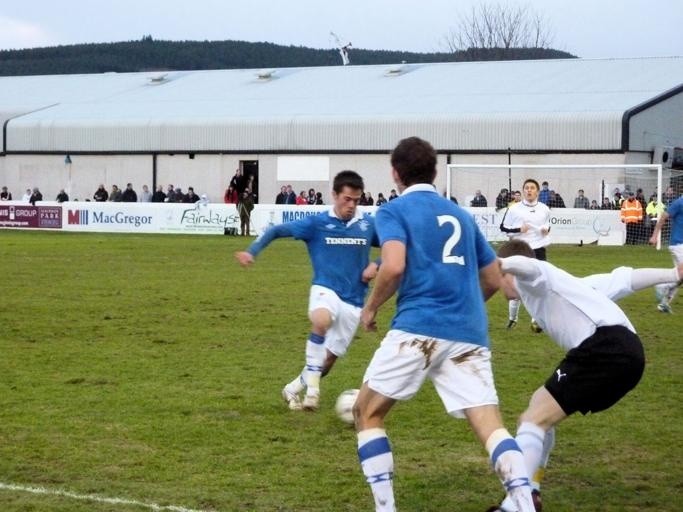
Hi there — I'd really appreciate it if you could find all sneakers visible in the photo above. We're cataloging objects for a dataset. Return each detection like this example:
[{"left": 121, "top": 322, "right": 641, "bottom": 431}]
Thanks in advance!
[
  {"left": 656, "top": 302, "right": 673, "bottom": 313},
  {"left": 486, "top": 488, "right": 543, "bottom": 512},
  {"left": 530, "top": 322, "right": 542, "bottom": 334},
  {"left": 281, "top": 383, "right": 303, "bottom": 411},
  {"left": 654, "top": 282, "right": 672, "bottom": 304},
  {"left": 506, "top": 320, "right": 517, "bottom": 330},
  {"left": 303, "top": 392, "right": 320, "bottom": 411}
]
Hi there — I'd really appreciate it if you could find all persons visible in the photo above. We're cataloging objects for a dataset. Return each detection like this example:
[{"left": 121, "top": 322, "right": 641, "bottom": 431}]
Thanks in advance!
[
  {"left": 495, "top": 237, "right": 682, "bottom": 486},
  {"left": 363, "top": 192, "right": 399, "bottom": 208},
  {"left": 236, "top": 171, "right": 384, "bottom": 412},
  {"left": 224, "top": 173, "right": 256, "bottom": 202},
  {"left": 525, "top": 181, "right": 672, "bottom": 247},
  {"left": 56, "top": 188, "right": 68, "bottom": 202},
  {"left": 275, "top": 185, "right": 324, "bottom": 203},
  {"left": 238, "top": 192, "right": 254, "bottom": 234},
  {"left": 94, "top": 182, "right": 209, "bottom": 203},
  {"left": 0, "top": 186, "right": 13, "bottom": 201},
  {"left": 22, "top": 188, "right": 42, "bottom": 204},
  {"left": 352, "top": 138, "right": 528, "bottom": 484},
  {"left": 449, "top": 189, "right": 523, "bottom": 209},
  {"left": 646, "top": 190, "right": 682, "bottom": 311},
  {"left": 502, "top": 178, "right": 551, "bottom": 335}
]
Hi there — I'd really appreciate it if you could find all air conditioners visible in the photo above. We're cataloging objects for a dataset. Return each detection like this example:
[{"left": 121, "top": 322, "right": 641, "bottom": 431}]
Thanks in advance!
[{"left": 652, "top": 144, "right": 673, "bottom": 170}]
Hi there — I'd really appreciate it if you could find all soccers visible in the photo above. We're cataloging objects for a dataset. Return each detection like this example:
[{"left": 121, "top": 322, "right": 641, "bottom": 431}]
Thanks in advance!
[{"left": 335, "top": 388, "right": 361, "bottom": 425}]
